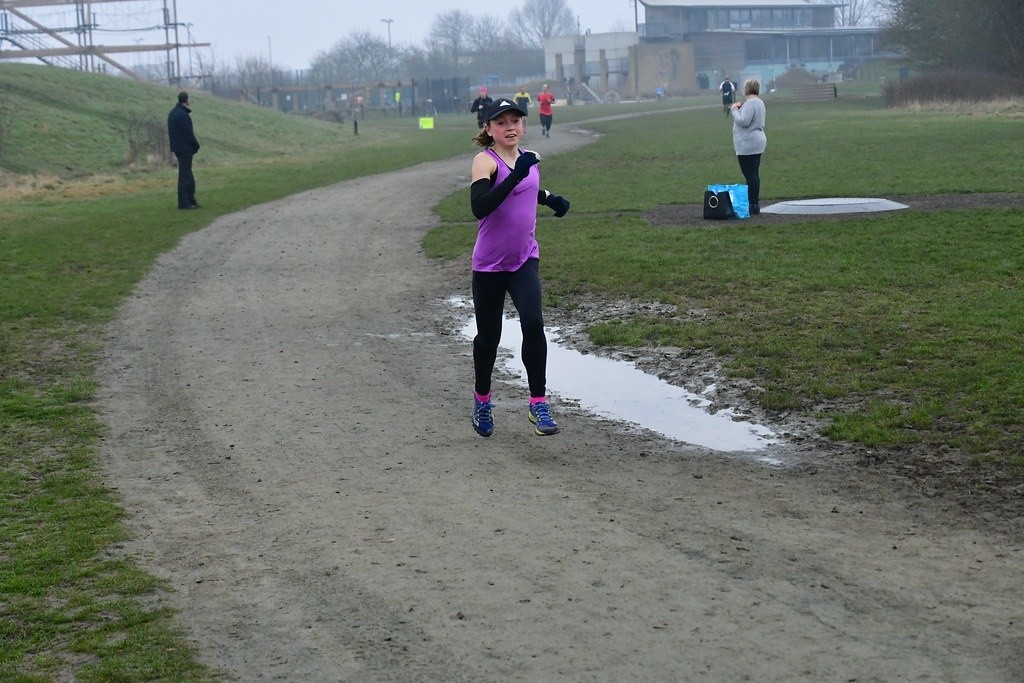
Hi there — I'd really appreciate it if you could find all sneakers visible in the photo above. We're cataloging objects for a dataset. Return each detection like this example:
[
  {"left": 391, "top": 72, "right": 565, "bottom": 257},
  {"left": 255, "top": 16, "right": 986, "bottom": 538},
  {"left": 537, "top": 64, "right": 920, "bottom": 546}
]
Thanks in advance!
[
  {"left": 528, "top": 398, "right": 560, "bottom": 436},
  {"left": 471, "top": 394, "right": 496, "bottom": 439}
]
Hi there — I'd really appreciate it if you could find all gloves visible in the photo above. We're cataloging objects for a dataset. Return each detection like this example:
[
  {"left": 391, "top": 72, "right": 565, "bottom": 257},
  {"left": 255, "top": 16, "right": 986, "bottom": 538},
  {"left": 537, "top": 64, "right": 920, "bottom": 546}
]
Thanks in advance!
[
  {"left": 545, "top": 193, "right": 570, "bottom": 219},
  {"left": 513, "top": 150, "right": 541, "bottom": 182}
]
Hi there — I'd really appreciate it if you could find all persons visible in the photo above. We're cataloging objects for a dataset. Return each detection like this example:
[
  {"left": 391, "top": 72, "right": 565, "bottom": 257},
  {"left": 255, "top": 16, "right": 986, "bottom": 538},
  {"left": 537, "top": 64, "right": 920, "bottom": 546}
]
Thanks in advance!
[
  {"left": 470, "top": 98, "right": 570, "bottom": 438},
  {"left": 719, "top": 75, "right": 735, "bottom": 117},
  {"left": 731, "top": 79, "right": 767, "bottom": 215},
  {"left": 656, "top": 84, "right": 662, "bottom": 102},
  {"left": 512, "top": 86, "right": 533, "bottom": 134},
  {"left": 394, "top": 90, "right": 401, "bottom": 105},
  {"left": 471, "top": 86, "right": 494, "bottom": 131},
  {"left": 168, "top": 91, "right": 201, "bottom": 210},
  {"left": 537, "top": 83, "right": 555, "bottom": 138},
  {"left": 730, "top": 78, "right": 738, "bottom": 103}
]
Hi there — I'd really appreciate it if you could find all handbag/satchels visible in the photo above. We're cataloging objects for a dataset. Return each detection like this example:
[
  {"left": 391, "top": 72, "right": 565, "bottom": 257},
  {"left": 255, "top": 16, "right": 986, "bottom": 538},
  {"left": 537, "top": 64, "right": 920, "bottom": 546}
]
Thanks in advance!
[
  {"left": 707, "top": 183, "right": 751, "bottom": 219},
  {"left": 703, "top": 190, "right": 736, "bottom": 220}
]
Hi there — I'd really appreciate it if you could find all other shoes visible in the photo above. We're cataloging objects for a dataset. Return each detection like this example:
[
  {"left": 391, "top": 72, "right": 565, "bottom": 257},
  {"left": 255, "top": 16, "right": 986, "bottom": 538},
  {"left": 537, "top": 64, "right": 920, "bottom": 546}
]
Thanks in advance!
[
  {"left": 183, "top": 201, "right": 202, "bottom": 209},
  {"left": 542, "top": 129, "right": 550, "bottom": 138},
  {"left": 723, "top": 110, "right": 730, "bottom": 118},
  {"left": 748, "top": 205, "right": 761, "bottom": 213}
]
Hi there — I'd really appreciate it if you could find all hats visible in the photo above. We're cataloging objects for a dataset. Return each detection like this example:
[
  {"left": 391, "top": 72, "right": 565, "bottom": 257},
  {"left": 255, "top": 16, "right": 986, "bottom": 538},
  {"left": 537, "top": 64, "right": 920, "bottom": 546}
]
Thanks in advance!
[
  {"left": 486, "top": 97, "right": 527, "bottom": 121},
  {"left": 479, "top": 87, "right": 488, "bottom": 92}
]
